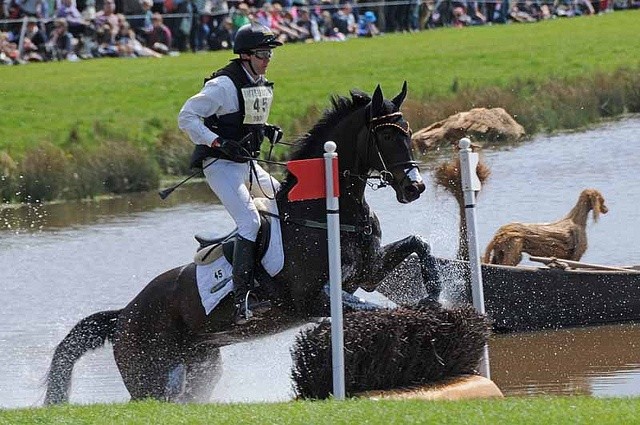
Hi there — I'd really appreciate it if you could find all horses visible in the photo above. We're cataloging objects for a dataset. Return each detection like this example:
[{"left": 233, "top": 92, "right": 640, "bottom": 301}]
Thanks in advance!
[{"left": 22, "top": 81, "right": 443, "bottom": 406}]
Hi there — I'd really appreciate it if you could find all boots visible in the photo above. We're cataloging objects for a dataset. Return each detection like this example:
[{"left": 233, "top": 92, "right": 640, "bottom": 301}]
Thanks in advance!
[{"left": 233, "top": 235, "right": 272, "bottom": 324}]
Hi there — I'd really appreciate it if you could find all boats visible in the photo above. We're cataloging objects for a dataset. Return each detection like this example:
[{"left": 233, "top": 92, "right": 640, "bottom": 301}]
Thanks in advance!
[{"left": 372, "top": 245, "right": 640, "bottom": 332}]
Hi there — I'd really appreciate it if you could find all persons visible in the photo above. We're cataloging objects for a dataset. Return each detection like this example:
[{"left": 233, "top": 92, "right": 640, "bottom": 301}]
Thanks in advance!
[
  {"left": 1, "top": 0, "right": 173, "bottom": 68},
  {"left": 176, "top": 22, "right": 287, "bottom": 325},
  {"left": 173, "top": 0, "right": 386, "bottom": 54},
  {"left": 389, "top": 0, "right": 640, "bottom": 33}
]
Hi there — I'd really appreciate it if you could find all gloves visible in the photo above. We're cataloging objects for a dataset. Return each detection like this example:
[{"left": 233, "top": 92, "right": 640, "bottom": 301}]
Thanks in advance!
[
  {"left": 265, "top": 124, "right": 284, "bottom": 146},
  {"left": 214, "top": 138, "right": 250, "bottom": 162}
]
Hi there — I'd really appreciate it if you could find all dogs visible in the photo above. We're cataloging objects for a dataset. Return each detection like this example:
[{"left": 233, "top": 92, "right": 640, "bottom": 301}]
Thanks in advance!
[{"left": 484, "top": 189, "right": 608, "bottom": 270}]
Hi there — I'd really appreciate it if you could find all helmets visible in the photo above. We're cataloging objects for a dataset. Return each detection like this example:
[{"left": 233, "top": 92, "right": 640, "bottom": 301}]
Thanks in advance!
[{"left": 233, "top": 22, "right": 283, "bottom": 55}]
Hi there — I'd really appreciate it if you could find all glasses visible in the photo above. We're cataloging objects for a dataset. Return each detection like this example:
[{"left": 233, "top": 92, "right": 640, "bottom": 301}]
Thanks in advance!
[{"left": 254, "top": 50, "right": 272, "bottom": 61}]
